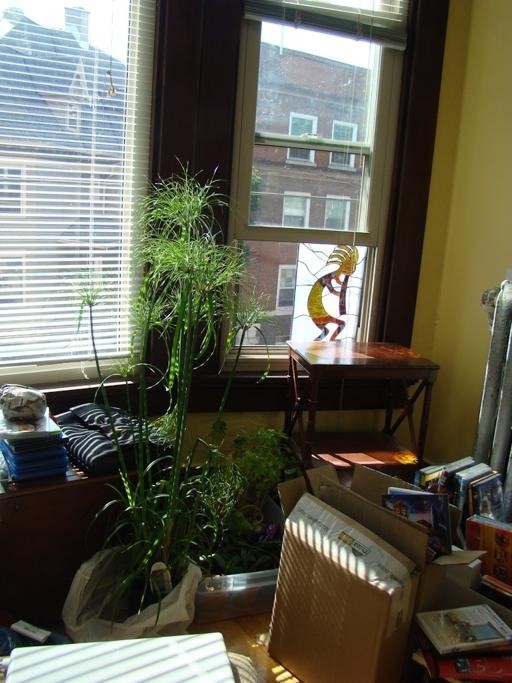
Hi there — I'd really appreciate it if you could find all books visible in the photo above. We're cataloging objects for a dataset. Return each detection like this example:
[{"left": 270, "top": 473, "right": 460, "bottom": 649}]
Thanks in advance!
[
  {"left": 467, "top": 514, "right": 512, "bottom": 584},
  {"left": 0, "top": 406, "right": 72, "bottom": 484},
  {"left": 387, "top": 487, "right": 435, "bottom": 497},
  {"left": 381, "top": 493, "right": 448, "bottom": 565},
  {"left": 414, "top": 603, "right": 511, "bottom": 656},
  {"left": 416, "top": 455, "right": 504, "bottom": 522},
  {"left": 480, "top": 574, "right": 512, "bottom": 609}
]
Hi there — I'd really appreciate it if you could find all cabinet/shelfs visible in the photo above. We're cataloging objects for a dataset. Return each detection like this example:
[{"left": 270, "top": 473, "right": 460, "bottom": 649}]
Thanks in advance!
[
  {"left": 0, "top": 465, "right": 146, "bottom": 627},
  {"left": 282, "top": 337, "right": 440, "bottom": 470}
]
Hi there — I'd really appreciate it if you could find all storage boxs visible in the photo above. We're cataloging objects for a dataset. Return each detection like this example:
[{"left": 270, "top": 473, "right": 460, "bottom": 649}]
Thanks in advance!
[
  {"left": 267, "top": 472, "right": 429, "bottom": 683},
  {"left": 305, "top": 463, "right": 460, "bottom": 625}
]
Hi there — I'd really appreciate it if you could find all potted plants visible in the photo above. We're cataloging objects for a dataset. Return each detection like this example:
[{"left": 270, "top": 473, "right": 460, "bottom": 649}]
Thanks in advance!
[{"left": 69, "top": 157, "right": 298, "bottom": 632}]
